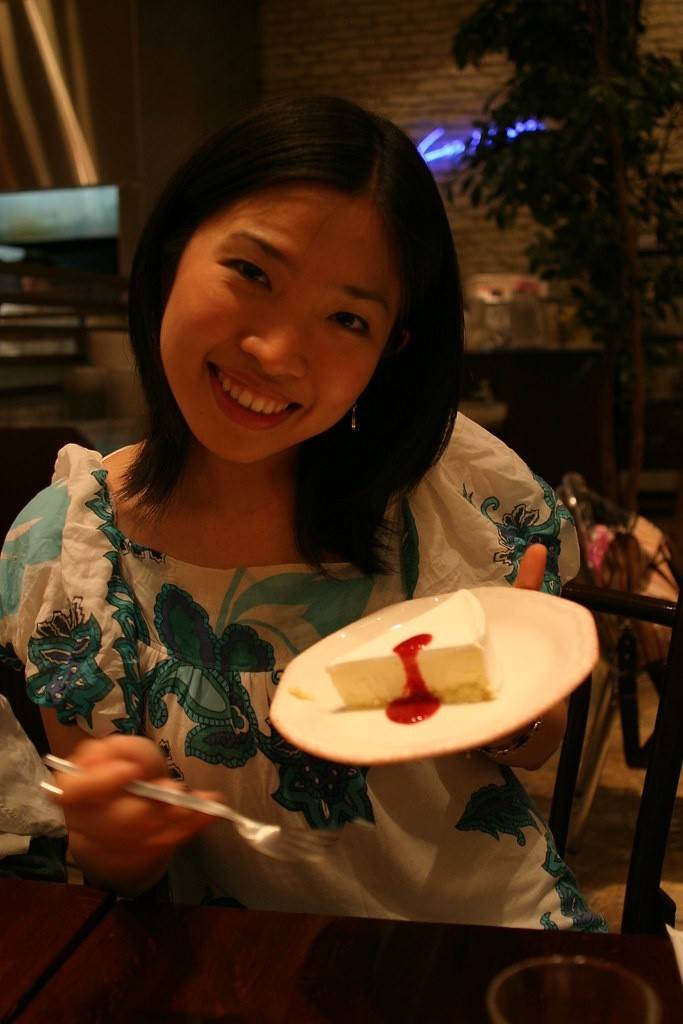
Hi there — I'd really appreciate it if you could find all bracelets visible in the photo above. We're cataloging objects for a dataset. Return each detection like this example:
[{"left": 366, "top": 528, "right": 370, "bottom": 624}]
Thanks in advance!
[{"left": 472, "top": 716, "right": 543, "bottom": 758}]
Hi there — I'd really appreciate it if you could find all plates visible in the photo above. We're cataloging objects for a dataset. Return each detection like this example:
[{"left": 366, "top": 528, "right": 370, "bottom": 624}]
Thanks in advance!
[{"left": 266, "top": 588, "right": 599, "bottom": 764}]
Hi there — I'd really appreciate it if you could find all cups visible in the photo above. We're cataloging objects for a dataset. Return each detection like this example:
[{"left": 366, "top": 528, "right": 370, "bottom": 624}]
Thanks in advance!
[{"left": 485, "top": 954, "right": 662, "bottom": 1024}]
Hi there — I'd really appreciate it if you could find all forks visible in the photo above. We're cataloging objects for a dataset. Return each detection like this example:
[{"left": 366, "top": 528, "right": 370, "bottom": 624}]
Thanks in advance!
[{"left": 41, "top": 749, "right": 341, "bottom": 865}]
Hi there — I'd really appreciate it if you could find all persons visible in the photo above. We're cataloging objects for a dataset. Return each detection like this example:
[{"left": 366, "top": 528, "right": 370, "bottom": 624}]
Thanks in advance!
[{"left": 0, "top": 93, "right": 613, "bottom": 934}]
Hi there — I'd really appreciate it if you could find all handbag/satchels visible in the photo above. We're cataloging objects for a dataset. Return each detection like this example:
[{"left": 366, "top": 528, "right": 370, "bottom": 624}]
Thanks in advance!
[{"left": 562, "top": 474, "right": 680, "bottom": 665}]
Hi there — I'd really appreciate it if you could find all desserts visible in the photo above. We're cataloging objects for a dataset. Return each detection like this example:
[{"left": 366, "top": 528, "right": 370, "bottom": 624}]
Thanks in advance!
[{"left": 325, "top": 587, "right": 495, "bottom": 708}]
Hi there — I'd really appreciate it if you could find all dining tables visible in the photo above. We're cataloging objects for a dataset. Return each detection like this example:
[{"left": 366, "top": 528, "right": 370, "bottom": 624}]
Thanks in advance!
[{"left": 0, "top": 876, "right": 683, "bottom": 1024}]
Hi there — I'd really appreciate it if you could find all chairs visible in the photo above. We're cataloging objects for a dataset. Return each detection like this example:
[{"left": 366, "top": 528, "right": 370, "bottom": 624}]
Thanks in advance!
[{"left": 548, "top": 583, "right": 683, "bottom": 932}]
